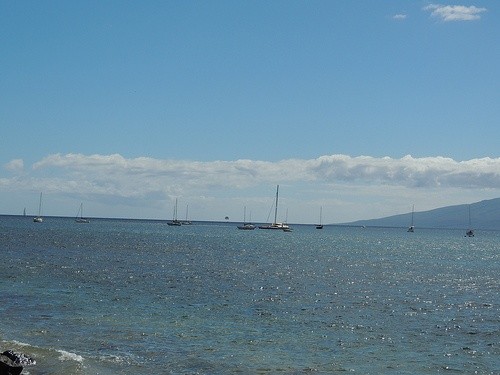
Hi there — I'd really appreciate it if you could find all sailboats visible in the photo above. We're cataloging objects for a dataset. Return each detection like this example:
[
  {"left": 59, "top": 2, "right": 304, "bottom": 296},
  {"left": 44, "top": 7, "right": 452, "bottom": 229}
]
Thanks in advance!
[
  {"left": 236, "top": 206, "right": 257, "bottom": 230},
  {"left": 258, "top": 184, "right": 292, "bottom": 232},
  {"left": 463, "top": 205, "right": 474, "bottom": 237},
  {"left": 315, "top": 206, "right": 323, "bottom": 229},
  {"left": 406, "top": 203, "right": 416, "bottom": 232},
  {"left": 33, "top": 192, "right": 44, "bottom": 223},
  {"left": 166, "top": 198, "right": 192, "bottom": 226},
  {"left": 71, "top": 202, "right": 89, "bottom": 223}
]
